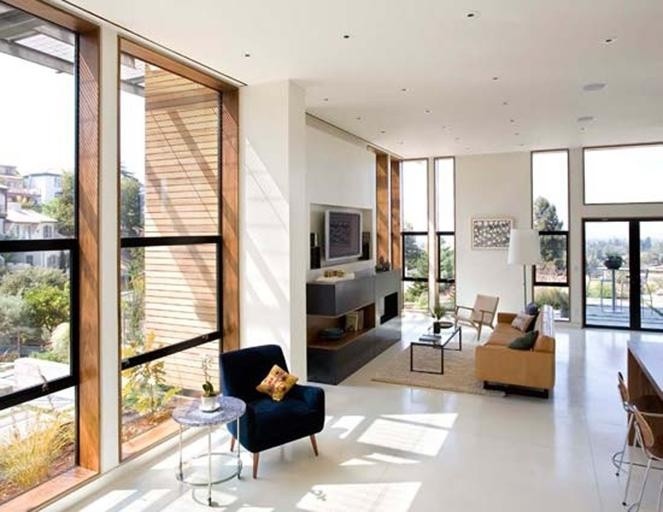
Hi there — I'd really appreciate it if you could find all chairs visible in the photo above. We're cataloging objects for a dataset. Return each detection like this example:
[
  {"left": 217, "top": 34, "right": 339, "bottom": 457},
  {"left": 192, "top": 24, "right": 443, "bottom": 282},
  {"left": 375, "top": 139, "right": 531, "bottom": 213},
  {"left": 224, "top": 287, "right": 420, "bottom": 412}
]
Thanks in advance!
[
  {"left": 451, "top": 294, "right": 500, "bottom": 341},
  {"left": 219, "top": 345, "right": 325, "bottom": 479},
  {"left": 612, "top": 371, "right": 663, "bottom": 512},
  {"left": 585, "top": 258, "right": 653, "bottom": 315}
]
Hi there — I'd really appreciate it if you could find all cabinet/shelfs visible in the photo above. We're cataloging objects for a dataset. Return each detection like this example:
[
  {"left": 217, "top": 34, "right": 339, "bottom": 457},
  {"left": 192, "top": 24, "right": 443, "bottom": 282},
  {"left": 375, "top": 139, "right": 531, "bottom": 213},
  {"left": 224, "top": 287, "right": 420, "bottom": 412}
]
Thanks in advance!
[{"left": 306, "top": 268, "right": 402, "bottom": 385}]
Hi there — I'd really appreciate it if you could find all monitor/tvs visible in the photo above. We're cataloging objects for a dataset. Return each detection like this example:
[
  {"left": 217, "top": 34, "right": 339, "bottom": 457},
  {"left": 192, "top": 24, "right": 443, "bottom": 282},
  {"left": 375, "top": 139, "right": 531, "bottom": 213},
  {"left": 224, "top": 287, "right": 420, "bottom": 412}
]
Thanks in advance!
[{"left": 323, "top": 206, "right": 363, "bottom": 262}]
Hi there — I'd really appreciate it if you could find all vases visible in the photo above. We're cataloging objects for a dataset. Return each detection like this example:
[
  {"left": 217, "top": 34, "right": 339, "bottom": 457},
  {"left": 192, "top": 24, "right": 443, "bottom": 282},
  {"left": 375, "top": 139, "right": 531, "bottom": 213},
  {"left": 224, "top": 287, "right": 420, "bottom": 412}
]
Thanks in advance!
[{"left": 201, "top": 396, "right": 220, "bottom": 412}]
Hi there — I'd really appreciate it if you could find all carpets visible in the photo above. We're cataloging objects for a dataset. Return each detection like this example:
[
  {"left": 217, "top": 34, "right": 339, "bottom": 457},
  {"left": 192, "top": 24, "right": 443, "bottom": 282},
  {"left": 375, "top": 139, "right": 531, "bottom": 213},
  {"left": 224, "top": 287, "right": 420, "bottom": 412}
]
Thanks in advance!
[{"left": 371, "top": 329, "right": 508, "bottom": 398}]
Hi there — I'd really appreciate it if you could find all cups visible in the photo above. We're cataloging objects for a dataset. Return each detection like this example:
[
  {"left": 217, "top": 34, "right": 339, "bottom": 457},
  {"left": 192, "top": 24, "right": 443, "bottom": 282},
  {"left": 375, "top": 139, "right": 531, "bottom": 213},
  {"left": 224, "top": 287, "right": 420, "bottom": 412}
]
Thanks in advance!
[{"left": 201, "top": 396, "right": 217, "bottom": 409}]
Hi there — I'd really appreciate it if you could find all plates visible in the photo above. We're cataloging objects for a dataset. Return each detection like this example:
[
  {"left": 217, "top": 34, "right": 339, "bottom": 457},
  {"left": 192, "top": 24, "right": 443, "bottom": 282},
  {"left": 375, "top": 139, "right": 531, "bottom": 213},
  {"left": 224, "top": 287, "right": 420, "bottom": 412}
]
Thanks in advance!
[{"left": 199, "top": 403, "right": 221, "bottom": 412}]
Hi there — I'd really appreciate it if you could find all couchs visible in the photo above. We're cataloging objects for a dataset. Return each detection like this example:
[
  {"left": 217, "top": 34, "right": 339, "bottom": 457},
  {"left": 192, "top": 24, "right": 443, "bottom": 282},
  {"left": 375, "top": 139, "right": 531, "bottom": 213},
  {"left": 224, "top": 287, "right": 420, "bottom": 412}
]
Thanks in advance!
[{"left": 473, "top": 304, "right": 555, "bottom": 398}]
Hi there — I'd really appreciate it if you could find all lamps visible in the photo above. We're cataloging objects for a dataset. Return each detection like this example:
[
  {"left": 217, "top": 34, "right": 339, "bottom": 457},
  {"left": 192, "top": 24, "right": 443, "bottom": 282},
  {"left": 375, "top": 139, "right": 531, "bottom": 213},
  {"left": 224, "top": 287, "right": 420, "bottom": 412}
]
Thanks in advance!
[{"left": 507, "top": 229, "right": 542, "bottom": 313}]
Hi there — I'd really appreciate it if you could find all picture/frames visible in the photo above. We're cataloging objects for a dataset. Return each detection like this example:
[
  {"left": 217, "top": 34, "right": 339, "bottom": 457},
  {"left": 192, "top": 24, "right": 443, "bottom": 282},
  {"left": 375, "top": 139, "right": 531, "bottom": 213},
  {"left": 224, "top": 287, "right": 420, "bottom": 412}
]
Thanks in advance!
[{"left": 471, "top": 217, "right": 514, "bottom": 251}]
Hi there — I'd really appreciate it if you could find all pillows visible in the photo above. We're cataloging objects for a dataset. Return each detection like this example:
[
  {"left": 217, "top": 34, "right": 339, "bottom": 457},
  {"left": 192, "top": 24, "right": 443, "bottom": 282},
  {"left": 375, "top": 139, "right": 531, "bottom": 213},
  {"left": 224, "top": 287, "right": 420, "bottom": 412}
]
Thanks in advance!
[
  {"left": 256, "top": 364, "right": 299, "bottom": 402},
  {"left": 508, "top": 302, "right": 539, "bottom": 350}
]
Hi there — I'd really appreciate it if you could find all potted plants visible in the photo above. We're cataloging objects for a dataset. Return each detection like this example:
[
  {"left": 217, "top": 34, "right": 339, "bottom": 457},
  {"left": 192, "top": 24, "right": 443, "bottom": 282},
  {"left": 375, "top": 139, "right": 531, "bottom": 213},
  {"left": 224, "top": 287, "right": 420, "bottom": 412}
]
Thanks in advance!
[
  {"left": 603, "top": 251, "right": 625, "bottom": 268},
  {"left": 430, "top": 300, "right": 445, "bottom": 333}
]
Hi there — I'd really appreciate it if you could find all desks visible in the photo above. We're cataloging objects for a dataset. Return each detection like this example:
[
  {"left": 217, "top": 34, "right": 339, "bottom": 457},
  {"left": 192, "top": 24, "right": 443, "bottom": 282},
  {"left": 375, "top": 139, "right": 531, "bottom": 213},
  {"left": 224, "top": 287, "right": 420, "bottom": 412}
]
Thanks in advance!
[
  {"left": 171, "top": 396, "right": 247, "bottom": 506},
  {"left": 627, "top": 340, "right": 663, "bottom": 447}
]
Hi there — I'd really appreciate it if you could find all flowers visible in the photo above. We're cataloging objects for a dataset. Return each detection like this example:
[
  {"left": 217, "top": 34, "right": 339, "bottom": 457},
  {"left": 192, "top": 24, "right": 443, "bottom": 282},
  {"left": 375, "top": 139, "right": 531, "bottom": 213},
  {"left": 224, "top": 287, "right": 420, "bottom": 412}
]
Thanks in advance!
[{"left": 201, "top": 354, "right": 220, "bottom": 396}]
